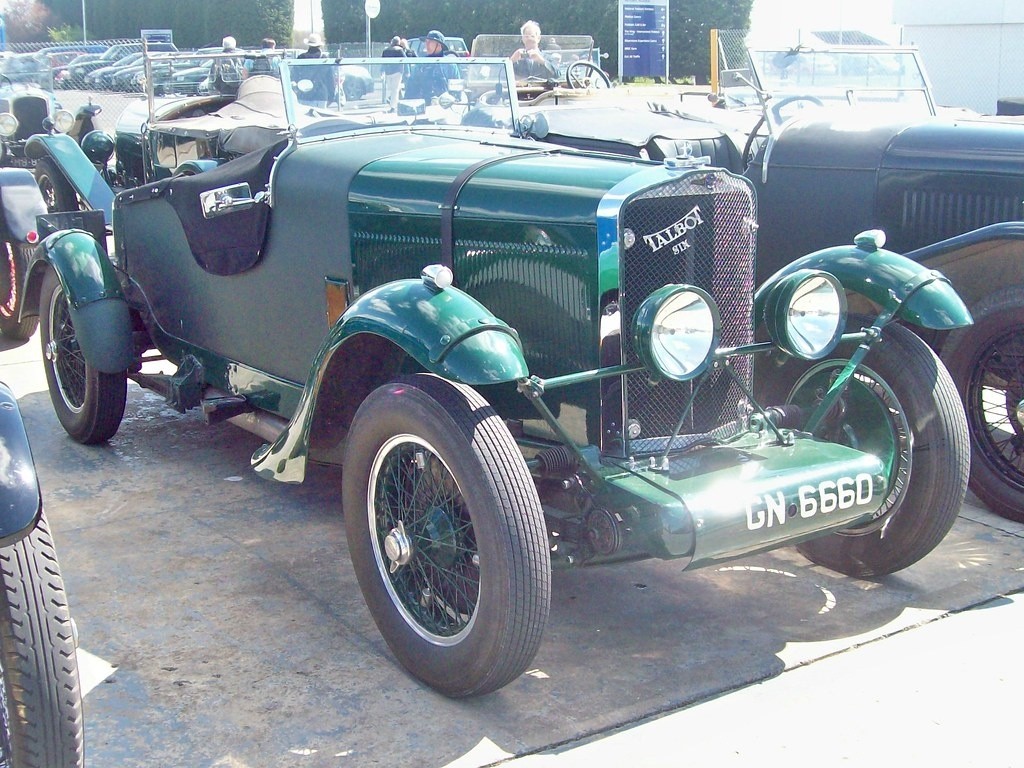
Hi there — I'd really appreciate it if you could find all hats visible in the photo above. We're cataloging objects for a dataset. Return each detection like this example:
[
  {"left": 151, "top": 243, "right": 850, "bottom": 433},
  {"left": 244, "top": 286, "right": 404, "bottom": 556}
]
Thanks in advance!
[
  {"left": 419, "top": 30, "right": 448, "bottom": 51},
  {"left": 303, "top": 34, "right": 325, "bottom": 46}
]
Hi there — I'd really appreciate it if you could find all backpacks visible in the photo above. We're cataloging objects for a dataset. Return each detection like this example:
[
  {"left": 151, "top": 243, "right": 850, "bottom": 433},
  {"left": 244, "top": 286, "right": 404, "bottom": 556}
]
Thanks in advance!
[
  {"left": 249, "top": 58, "right": 276, "bottom": 76},
  {"left": 213, "top": 57, "right": 242, "bottom": 92}
]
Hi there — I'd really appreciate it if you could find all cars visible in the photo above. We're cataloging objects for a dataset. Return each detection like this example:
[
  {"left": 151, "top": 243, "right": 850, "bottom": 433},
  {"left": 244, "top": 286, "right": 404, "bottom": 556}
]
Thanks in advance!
[
  {"left": 468, "top": 44, "right": 1024, "bottom": 524},
  {"left": 172, "top": 49, "right": 259, "bottom": 97},
  {"left": 46, "top": 52, "right": 108, "bottom": 86},
  {"left": 0, "top": 56, "right": 76, "bottom": 167},
  {"left": 0, "top": 112, "right": 49, "bottom": 340},
  {"left": 465, "top": 33, "right": 613, "bottom": 134},
  {"left": 84, "top": 50, "right": 203, "bottom": 97},
  {"left": 259, "top": 49, "right": 375, "bottom": 101},
  {"left": 15, "top": 33, "right": 974, "bottom": 698},
  {"left": 0, "top": 381, "right": 86, "bottom": 768}
]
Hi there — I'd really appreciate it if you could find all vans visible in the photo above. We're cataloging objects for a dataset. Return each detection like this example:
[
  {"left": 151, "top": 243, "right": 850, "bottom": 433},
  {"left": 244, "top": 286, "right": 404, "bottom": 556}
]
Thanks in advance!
[
  {"left": 33, "top": 46, "right": 108, "bottom": 56},
  {"left": 403, "top": 37, "right": 470, "bottom": 80}
]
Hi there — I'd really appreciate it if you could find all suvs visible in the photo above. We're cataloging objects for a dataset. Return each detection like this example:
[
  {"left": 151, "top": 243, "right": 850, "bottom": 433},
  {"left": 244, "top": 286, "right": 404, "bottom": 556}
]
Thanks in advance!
[{"left": 67, "top": 43, "right": 180, "bottom": 92}]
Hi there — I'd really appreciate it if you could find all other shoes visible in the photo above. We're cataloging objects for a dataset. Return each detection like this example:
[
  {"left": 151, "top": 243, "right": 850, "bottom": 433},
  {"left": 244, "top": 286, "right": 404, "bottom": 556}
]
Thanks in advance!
[{"left": 388, "top": 109, "right": 396, "bottom": 113}]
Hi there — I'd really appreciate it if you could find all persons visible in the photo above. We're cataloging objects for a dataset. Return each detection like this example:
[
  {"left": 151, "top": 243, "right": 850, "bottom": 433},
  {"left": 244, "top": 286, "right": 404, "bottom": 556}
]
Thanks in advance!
[
  {"left": 380, "top": 36, "right": 410, "bottom": 113},
  {"left": 400, "top": 38, "right": 420, "bottom": 90},
  {"left": 545, "top": 37, "right": 598, "bottom": 72},
  {"left": 404, "top": 29, "right": 462, "bottom": 106},
  {"left": 241, "top": 37, "right": 286, "bottom": 82},
  {"left": 510, "top": 20, "right": 562, "bottom": 81},
  {"left": 210, "top": 36, "right": 250, "bottom": 96},
  {"left": 290, "top": 33, "right": 335, "bottom": 110}
]
